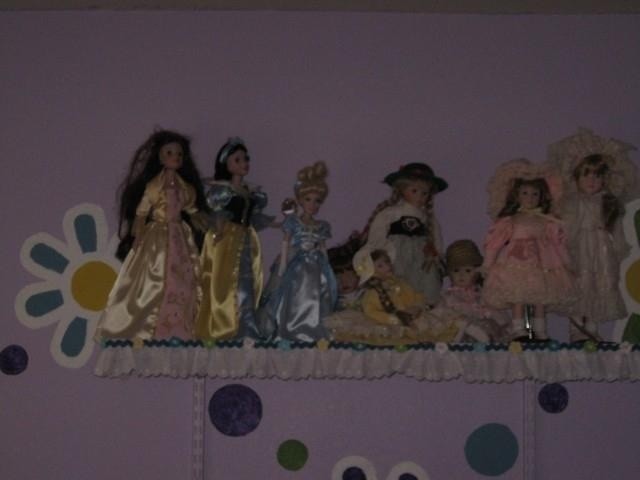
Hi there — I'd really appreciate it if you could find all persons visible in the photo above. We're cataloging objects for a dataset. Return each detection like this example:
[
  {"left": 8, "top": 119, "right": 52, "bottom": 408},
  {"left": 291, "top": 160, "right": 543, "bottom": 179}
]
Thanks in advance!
[{"left": 96, "top": 125, "right": 634, "bottom": 347}]
